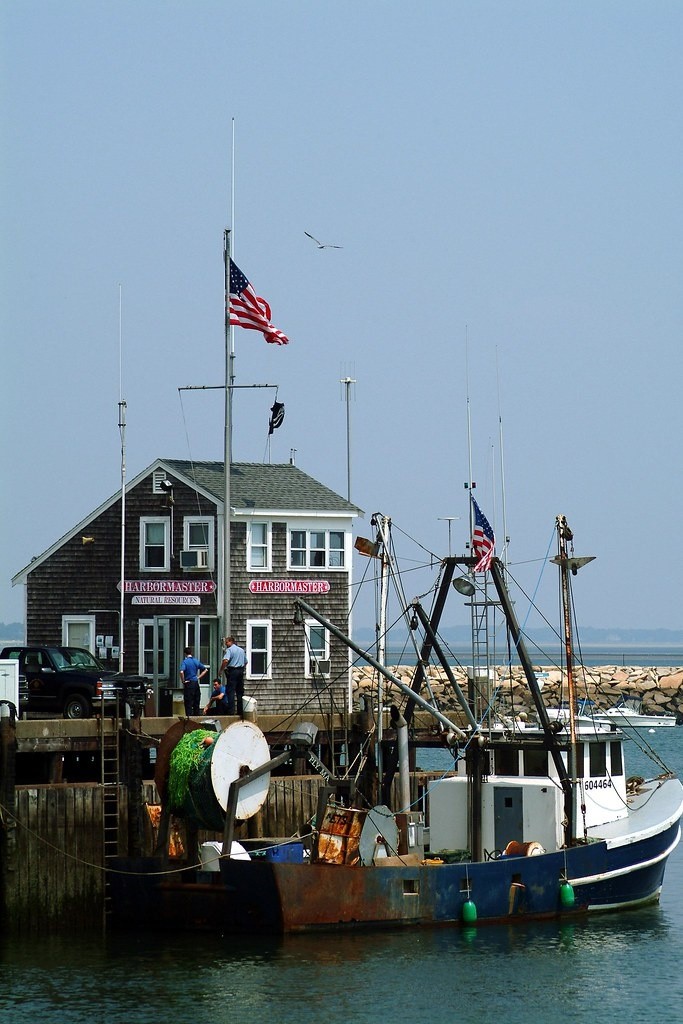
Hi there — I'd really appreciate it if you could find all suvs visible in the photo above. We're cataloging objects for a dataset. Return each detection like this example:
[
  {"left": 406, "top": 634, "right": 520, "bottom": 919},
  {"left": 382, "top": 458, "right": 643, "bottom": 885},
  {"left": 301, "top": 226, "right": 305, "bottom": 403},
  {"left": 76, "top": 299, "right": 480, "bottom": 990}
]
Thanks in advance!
[{"left": 0, "top": 645, "right": 155, "bottom": 718}]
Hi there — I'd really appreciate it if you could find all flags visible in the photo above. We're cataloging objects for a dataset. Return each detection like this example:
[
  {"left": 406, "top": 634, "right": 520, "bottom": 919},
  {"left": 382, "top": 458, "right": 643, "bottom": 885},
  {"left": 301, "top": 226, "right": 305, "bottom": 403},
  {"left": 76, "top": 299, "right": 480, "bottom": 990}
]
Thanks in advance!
[
  {"left": 223, "top": 237, "right": 289, "bottom": 346},
  {"left": 270, "top": 401, "right": 286, "bottom": 437},
  {"left": 468, "top": 491, "right": 496, "bottom": 572}
]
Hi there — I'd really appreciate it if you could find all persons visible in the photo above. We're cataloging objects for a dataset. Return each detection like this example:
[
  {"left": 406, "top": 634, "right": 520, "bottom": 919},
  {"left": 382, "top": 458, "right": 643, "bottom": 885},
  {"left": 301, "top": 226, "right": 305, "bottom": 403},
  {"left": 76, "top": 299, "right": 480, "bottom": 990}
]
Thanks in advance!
[
  {"left": 203, "top": 679, "right": 233, "bottom": 716},
  {"left": 179, "top": 648, "right": 208, "bottom": 718},
  {"left": 218, "top": 636, "right": 248, "bottom": 715}
]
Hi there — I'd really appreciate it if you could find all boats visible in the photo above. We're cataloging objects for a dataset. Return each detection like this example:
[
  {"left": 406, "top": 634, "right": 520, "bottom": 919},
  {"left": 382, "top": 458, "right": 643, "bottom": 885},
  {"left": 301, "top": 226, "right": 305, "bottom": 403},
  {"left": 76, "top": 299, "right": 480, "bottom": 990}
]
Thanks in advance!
[
  {"left": 590, "top": 706, "right": 679, "bottom": 727},
  {"left": 459, "top": 707, "right": 621, "bottom": 736},
  {"left": 110, "top": 553, "right": 683, "bottom": 936}
]
